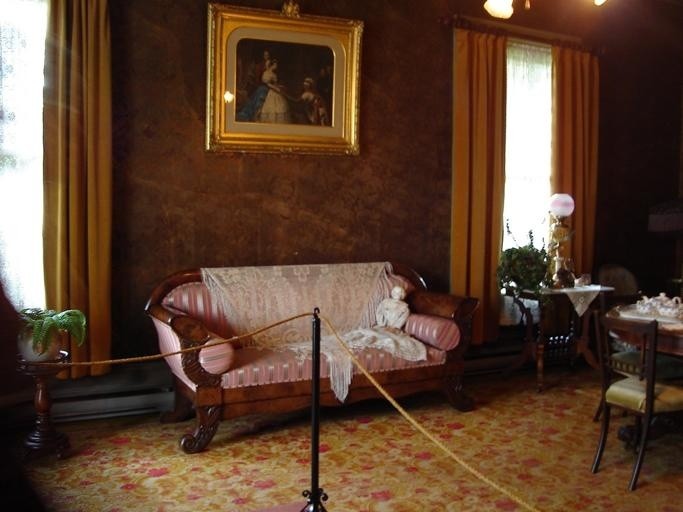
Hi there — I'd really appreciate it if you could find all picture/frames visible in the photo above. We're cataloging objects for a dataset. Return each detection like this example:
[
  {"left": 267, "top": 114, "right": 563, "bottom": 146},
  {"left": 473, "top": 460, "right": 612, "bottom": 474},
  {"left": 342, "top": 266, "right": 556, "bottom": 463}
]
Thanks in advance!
[{"left": 203, "top": 1, "right": 365, "bottom": 158}]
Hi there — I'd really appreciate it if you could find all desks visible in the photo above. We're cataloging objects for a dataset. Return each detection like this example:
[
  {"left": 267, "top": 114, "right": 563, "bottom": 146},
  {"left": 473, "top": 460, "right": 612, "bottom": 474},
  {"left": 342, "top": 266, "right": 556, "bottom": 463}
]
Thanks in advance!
[
  {"left": 493, "top": 281, "right": 615, "bottom": 394},
  {"left": 598, "top": 298, "right": 683, "bottom": 455},
  {"left": 10, "top": 349, "right": 73, "bottom": 462}
]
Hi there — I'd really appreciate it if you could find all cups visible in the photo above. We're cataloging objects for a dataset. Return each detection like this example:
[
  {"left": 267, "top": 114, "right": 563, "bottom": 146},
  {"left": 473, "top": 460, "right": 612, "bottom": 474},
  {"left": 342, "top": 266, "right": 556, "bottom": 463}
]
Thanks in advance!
[{"left": 637, "top": 300, "right": 681, "bottom": 317}]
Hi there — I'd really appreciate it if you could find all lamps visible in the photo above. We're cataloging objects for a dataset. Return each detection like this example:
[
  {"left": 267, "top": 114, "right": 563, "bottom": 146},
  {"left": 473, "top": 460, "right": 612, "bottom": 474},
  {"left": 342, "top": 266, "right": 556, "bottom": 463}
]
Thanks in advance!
[{"left": 545, "top": 193, "right": 574, "bottom": 284}]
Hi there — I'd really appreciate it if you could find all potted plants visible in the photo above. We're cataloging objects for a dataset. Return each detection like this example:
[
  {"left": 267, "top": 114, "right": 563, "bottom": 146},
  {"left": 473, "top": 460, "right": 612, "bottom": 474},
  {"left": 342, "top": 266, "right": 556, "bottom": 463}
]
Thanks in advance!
[
  {"left": 493, "top": 215, "right": 576, "bottom": 312},
  {"left": 14, "top": 306, "right": 86, "bottom": 363}
]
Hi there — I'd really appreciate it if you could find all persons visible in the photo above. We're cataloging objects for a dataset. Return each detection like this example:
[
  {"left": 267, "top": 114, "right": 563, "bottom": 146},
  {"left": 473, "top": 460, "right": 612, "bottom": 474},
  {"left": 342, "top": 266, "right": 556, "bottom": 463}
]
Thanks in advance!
[
  {"left": 343, "top": 285, "right": 428, "bottom": 361},
  {"left": 237, "top": 49, "right": 333, "bottom": 126}
]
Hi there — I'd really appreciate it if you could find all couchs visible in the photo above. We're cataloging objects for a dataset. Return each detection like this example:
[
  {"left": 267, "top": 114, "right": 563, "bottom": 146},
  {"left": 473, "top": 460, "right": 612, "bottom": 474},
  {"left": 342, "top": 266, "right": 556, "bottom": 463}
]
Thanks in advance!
[{"left": 141, "top": 258, "right": 483, "bottom": 454}]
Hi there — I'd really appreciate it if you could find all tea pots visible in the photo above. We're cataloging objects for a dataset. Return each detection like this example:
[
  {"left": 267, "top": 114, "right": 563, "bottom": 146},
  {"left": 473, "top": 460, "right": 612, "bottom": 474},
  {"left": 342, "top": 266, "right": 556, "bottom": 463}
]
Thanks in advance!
[{"left": 641, "top": 291, "right": 681, "bottom": 317}]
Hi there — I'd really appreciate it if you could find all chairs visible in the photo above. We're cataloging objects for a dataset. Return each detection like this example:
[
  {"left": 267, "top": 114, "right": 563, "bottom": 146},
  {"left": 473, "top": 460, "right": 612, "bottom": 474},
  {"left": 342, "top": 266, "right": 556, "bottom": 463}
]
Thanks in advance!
[
  {"left": 587, "top": 307, "right": 683, "bottom": 492},
  {"left": 590, "top": 261, "right": 683, "bottom": 425}
]
